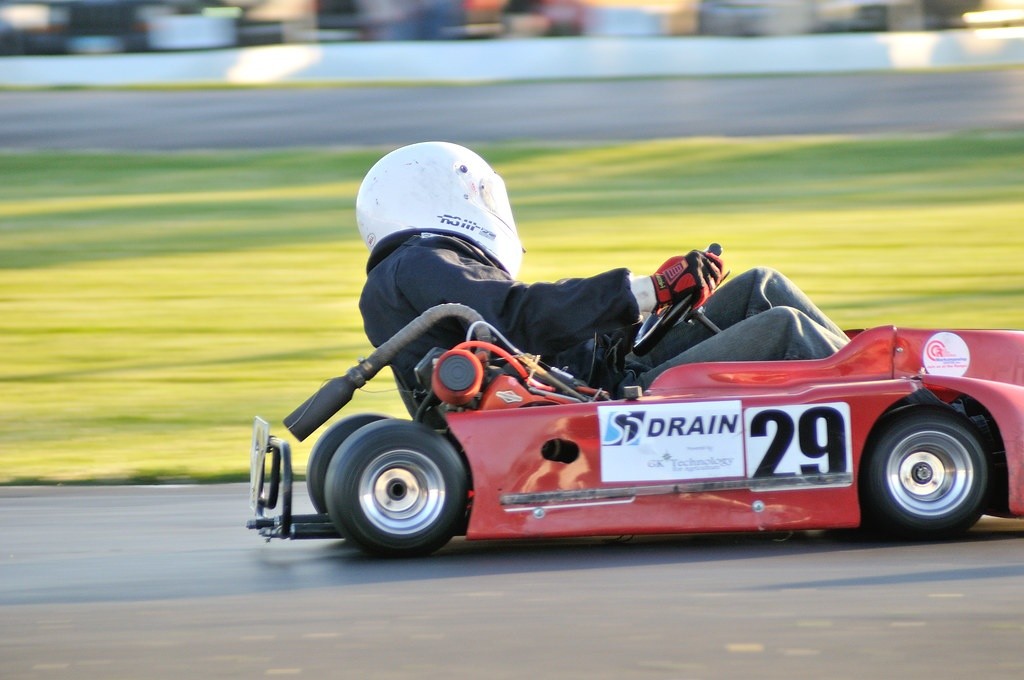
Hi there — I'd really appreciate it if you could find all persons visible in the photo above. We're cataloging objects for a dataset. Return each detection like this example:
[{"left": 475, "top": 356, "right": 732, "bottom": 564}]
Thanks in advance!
[{"left": 355, "top": 141, "right": 851, "bottom": 425}]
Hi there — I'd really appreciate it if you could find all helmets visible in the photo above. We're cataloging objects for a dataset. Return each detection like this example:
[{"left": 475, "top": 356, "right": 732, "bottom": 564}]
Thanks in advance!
[{"left": 356, "top": 141, "right": 522, "bottom": 282}]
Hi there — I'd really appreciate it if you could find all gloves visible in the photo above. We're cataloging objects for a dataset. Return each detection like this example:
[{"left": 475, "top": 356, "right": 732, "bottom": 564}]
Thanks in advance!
[{"left": 651, "top": 249, "right": 723, "bottom": 322}]
